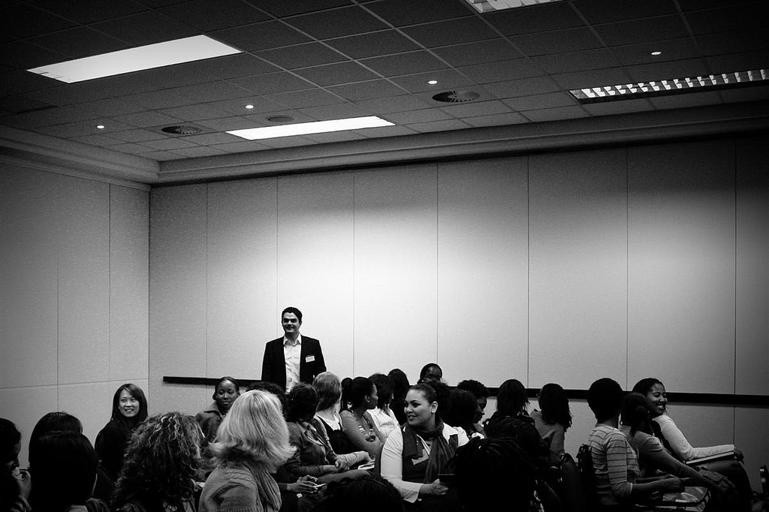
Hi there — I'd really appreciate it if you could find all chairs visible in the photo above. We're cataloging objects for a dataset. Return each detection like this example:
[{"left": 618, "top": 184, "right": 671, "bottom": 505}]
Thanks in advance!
[
  {"left": 628, "top": 434, "right": 693, "bottom": 482},
  {"left": 548, "top": 466, "right": 596, "bottom": 511},
  {"left": 653, "top": 421, "right": 741, "bottom": 464},
  {"left": 576, "top": 442, "right": 683, "bottom": 504},
  {"left": 530, "top": 472, "right": 571, "bottom": 511}
]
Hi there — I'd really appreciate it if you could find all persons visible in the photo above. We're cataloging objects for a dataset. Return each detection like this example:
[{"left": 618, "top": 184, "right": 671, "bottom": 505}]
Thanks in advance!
[
  {"left": 1, "top": 364, "right": 489, "bottom": 512},
  {"left": 262, "top": 306, "right": 327, "bottom": 394},
  {"left": 484, "top": 377, "right": 755, "bottom": 510}
]
